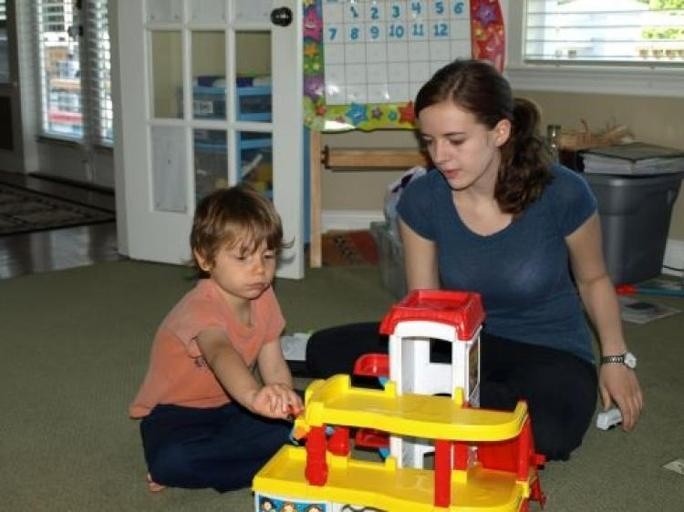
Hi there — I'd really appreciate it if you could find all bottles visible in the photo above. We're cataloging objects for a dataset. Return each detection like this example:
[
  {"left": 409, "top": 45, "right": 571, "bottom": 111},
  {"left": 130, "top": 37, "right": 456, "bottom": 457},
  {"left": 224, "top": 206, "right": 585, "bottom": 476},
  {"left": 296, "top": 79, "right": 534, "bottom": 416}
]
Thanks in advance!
[{"left": 546, "top": 124, "right": 562, "bottom": 164}]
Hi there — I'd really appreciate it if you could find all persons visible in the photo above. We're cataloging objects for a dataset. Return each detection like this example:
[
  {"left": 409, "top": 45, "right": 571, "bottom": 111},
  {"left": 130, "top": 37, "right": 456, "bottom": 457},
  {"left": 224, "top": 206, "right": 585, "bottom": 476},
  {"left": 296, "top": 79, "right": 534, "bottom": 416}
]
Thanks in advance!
[
  {"left": 308, "top": 60, "right": 642, "bottom": 460},
  {"left": 128, "top": 184, "right": 305, "bottom": 492}
]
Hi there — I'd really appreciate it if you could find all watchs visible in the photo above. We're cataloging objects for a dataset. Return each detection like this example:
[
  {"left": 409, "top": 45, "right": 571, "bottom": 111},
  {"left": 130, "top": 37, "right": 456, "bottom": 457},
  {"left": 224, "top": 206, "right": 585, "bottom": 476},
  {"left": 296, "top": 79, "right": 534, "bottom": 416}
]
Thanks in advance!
[{"left": 600, "top": 351, "right": 637, "bottom": 370}]
